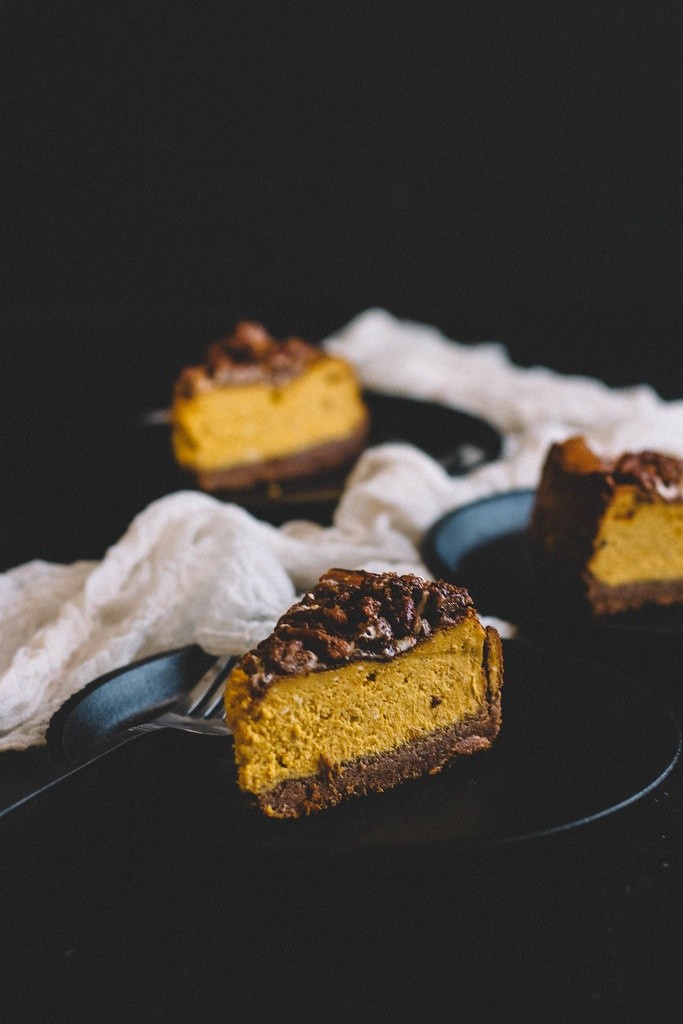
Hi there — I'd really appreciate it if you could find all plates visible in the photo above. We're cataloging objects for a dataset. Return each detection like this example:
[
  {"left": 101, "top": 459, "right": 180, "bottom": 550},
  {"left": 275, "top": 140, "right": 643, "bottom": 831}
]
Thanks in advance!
[
  {"left": 420, "top": 490, "right": 683, "bottom": 639},
  {"left": 47, "top": 640, "right": 683, "bottom": 847},
  {"left": 116, "top": 388, "right": 504, "bottom": 528}
]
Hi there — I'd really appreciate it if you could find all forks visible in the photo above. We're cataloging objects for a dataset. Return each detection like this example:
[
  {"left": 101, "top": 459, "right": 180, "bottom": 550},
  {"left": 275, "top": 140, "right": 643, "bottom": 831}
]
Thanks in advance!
[{"left": 1, "top": 654, "right": 232, "bottom": 817}]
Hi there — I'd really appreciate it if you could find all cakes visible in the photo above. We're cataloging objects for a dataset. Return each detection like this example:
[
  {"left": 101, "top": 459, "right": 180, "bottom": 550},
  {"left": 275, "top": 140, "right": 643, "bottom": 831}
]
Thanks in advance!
[
  {"left": 223, "top": 568, "right": 502, "bottom": 823},
  {"left": 170, "top": 323, "right": 367, "bottom": 490},
  {"left": 523, "top": 435, "right": 683, "bottom": 614}
]
